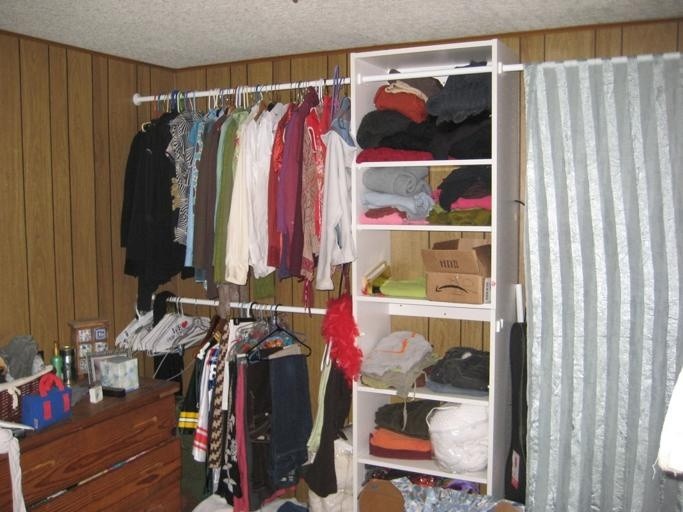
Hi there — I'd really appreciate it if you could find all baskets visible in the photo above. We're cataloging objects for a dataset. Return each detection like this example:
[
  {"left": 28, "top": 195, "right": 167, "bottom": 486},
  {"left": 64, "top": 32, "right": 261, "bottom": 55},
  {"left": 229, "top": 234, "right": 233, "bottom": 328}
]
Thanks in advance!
[{"left": 0, "top": 364, "right": 57, "bottom": 434}]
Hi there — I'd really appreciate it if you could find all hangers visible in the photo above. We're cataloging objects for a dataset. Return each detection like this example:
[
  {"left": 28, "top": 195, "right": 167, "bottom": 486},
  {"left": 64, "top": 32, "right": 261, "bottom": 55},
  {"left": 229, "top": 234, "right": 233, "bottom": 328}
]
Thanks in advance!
[
  {"left": 115, "top": 292, "right": 341, "bottom": 382},
  {"left": 141, "top": 65, "right": 352, "bottom": 129}
]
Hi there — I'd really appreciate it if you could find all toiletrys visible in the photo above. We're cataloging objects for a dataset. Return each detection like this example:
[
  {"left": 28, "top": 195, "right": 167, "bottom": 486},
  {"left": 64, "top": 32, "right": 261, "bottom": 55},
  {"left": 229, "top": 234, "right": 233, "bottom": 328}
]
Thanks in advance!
[{"left": 50, "top": 340, "right": 64, "bottom": 382}]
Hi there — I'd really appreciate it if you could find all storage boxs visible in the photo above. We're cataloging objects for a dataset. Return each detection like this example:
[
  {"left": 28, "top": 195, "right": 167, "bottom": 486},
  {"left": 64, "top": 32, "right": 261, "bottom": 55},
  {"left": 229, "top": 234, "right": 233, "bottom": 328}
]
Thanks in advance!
[
  {"left": 423, "top": 238, "right": 492, "bottom": 274},
  {"left": 427, "top": 272, "right": 491, "bottom": 305},
  {"left": 97, "top": 357, "right": 139, "bottom": 392}
]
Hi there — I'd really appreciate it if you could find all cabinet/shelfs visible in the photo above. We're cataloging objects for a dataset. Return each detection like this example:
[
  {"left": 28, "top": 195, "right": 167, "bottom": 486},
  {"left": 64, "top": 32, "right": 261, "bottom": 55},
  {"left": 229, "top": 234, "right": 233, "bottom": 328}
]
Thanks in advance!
[
  {"left": 351, "top": 38, "right": 520, "bottom": 512},
  {"left": 0, "top": 379, "right": 182, "bottom": 512}
]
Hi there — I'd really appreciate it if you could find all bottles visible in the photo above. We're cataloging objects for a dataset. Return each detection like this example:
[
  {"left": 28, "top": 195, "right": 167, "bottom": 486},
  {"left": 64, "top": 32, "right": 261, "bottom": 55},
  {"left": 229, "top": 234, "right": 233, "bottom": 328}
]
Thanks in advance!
[{"left": 51, "top": 340, "right": 64, "bottom": 381}]
